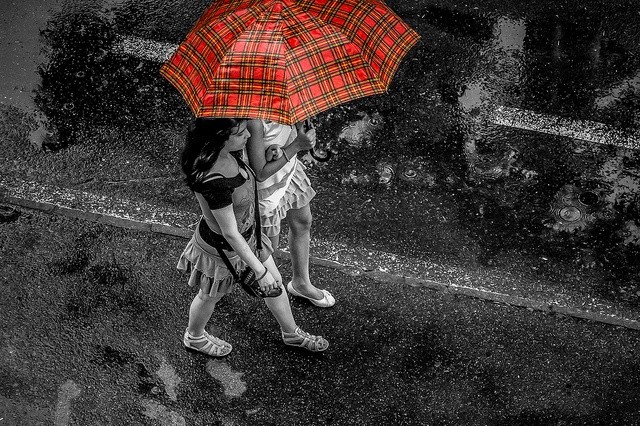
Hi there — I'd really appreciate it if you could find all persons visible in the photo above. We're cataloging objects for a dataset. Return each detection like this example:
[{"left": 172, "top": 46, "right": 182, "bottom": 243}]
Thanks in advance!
[
  {"left": 244, "top": 115, "right": 337, "bottom": 308},
  {"left": 172, "top": 117, "right": 331, "bottom": 360}
]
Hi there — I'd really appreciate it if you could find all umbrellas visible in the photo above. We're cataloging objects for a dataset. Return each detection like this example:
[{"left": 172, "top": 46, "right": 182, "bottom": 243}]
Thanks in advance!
[{"left": 157, "top": 0, "right": 422, "bottom": 127}]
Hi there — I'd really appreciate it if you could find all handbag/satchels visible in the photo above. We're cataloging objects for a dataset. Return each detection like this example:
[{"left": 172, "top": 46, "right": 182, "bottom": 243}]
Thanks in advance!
[{"left": 241, "top": 253, "right": 269, "bottom": 298}]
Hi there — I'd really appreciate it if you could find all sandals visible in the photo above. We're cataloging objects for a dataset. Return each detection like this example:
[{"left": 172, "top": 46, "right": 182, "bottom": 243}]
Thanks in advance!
[
  {"left": 286, "top": 281, "right": 335, "bottom": 309},
  {"left": 281, "top": 327, "right": 329, "bottom": 352},
  {"left": 183, "top": 327, "right": 232, "bottom": 357}
]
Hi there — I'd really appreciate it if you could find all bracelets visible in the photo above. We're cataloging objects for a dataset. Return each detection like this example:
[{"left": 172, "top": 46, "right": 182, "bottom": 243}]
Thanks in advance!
[
  {"left": 252, "top": 266, "right": 269, "bottom": 282},
  {"left": 281, "top": 147, "right": 293, "bottom": 165}
]
